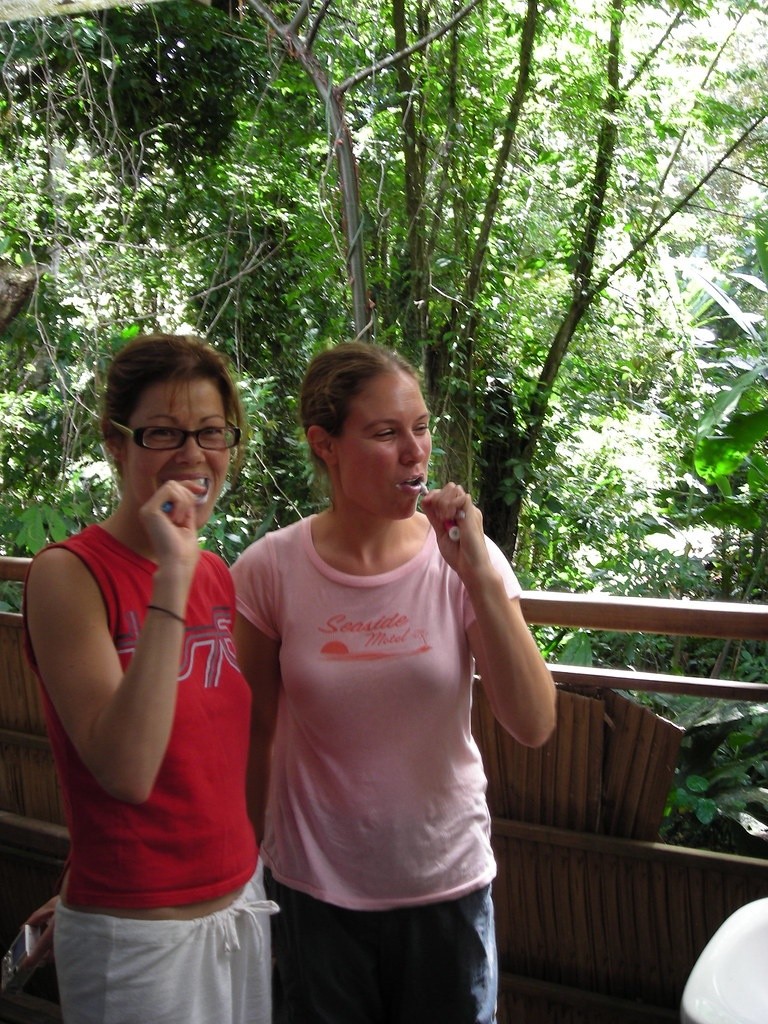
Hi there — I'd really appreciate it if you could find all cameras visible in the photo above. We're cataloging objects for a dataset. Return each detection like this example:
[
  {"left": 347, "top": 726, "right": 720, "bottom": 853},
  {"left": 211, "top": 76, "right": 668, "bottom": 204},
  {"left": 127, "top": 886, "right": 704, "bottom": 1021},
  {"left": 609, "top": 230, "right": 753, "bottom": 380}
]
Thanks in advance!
[{"left": 0, "top": 923, "right": 43, "bottom": 998}]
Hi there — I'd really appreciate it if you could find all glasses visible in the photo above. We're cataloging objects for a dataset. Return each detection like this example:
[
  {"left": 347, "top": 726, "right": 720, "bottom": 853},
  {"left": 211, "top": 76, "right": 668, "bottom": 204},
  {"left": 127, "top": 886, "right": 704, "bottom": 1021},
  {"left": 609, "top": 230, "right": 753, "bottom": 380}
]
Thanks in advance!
[{"left": 109, "top": 418, "right": 243, "bottom": 451}]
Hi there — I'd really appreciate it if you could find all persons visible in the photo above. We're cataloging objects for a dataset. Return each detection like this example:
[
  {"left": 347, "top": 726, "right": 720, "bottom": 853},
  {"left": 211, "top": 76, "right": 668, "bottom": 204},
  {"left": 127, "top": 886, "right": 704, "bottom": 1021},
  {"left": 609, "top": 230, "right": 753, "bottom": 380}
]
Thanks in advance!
[
  {"left": 229, "top": 342, "right": 557, "bottom": 1024},
  {"left": 22, "top": 333, "right": 280, "bottom": 1024}
]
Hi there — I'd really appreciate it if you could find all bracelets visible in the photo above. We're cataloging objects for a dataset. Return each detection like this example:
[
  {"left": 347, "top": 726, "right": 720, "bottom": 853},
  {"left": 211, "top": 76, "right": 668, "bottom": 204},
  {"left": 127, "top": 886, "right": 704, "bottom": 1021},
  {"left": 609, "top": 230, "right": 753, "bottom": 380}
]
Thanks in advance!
[{"left": 148, "top": 605, "right": 184, "bottom": 624}]
